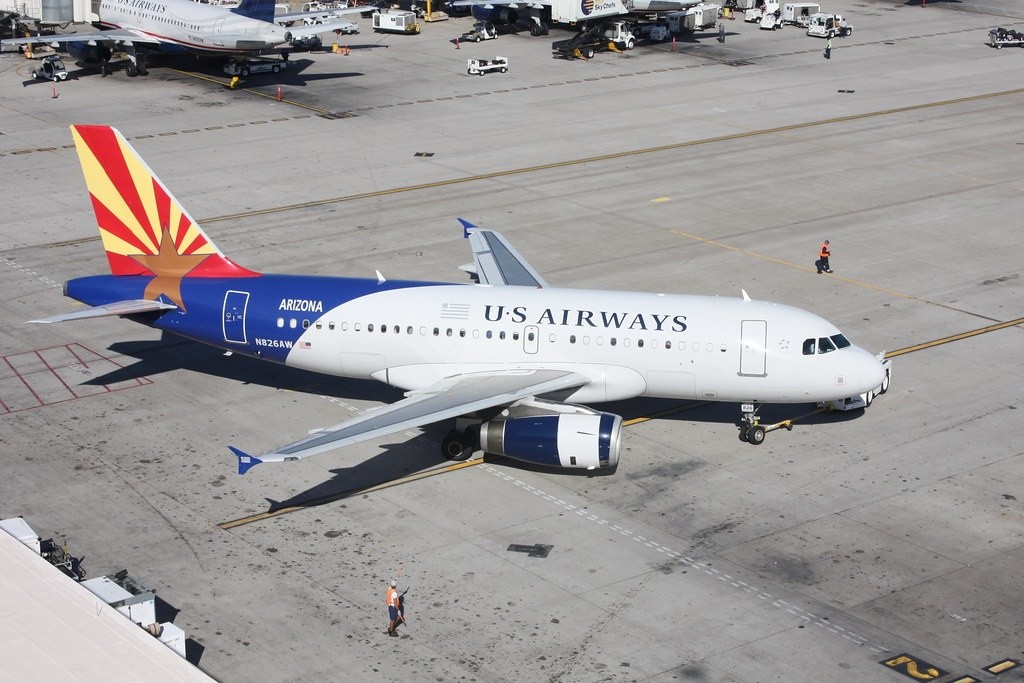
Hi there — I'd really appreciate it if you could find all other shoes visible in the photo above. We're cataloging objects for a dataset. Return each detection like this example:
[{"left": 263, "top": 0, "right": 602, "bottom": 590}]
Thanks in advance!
[
  {"left": 818, "top": 270, "right": 822, "bottom": 274},
  {"left": 390, "top": 629, "right": 399, "bottom": 637},
  {"left": 827, "top": 270, "right": 833, "bottom": 273}
]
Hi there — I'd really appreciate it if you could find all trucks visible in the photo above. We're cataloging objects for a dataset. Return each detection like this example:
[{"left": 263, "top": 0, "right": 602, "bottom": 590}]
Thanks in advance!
[{"left": 807, "top": 13, "right": 853, "bottom": 37}]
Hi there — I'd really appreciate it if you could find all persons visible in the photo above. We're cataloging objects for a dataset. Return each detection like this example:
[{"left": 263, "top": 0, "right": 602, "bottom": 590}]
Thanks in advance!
[
  {"left": 840, "top": 18, "right": 847, "bottom": 38},
  {"left": 386, "top": 577, "right": 401, "bottom": 637},
  {"left": 718, "top": 23, "right": 725, "bottom": 43},
  {"left": 818, "top": 240, "right": 833, "bottom": 274},
  {"left": 825, "top": 37, "right": 832, "bottom": 59},
  {"left": 760, "top": 2, "right": 781, "bottom": 22}
]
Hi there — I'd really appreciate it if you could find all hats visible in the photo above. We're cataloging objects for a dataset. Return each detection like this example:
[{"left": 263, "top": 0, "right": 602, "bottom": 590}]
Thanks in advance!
[{"left": 390, "top": 581, "right": 396, "bottom": 587}]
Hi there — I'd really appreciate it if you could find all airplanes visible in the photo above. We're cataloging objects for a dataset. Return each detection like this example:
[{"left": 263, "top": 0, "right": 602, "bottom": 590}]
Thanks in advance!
[
  {"left": 22, "top": 124, "right": 889, "bottom": 472},
  {"left": 0, "top": 0, "right": 380, "bottom": 77}
]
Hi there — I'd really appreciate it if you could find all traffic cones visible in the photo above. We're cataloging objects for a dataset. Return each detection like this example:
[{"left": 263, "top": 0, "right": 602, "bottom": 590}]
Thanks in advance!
[
  {"left": 278, "top": 83, "right": 283, "bottom": 102},
  {"left": 51, "top": 81, "right": 58, "bottom": 98},
  {"left": 454, "top": 36, "right": 462, "bottom": 48},
  {"left": 670, "top": 34, "right": 677, "bottom": 52}
]
